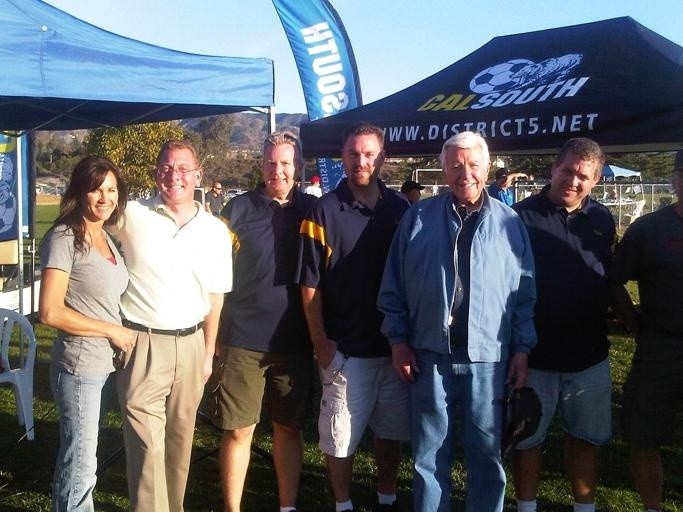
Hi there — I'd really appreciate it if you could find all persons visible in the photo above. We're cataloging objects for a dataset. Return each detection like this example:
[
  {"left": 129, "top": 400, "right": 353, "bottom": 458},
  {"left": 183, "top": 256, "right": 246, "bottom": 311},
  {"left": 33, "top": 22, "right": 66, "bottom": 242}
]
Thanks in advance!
[
  {"left": 377, "top": 132, "right": 536, "bottom": 512},
  {"left": 303, "top": 176, "right": 322, "bottom": 197},
  {"left": 38, "top": 156, "right": 137, "bottom": 511},
  {"left": 205, "top": 182, "right": 226, "bottom": 216},
  {"left": 523, "top": 175, "right": 537, "bottom": 200},
  {"left": 610, "top": 148, "right": 682, "bottom": 512},
  {"left": 401, "top": 180, "right": 426, "bottom": 204},
  {"left": 103, "top": 140, "right": 232, "bottom": 512},
  {"left": 489, "top": 168, "right": 528, "bottom": 206},
  {"left": 213, "top": 131, "right": 323, "bottom": 511},
  {"left": 295, "top": 124, "right": 413, "bottom": 511},
  {"left": 431, "top": 180, "right": 439, "bottom": 195},
  {"left": 508, "top": 137, "right": 619, "bottom": 512}
]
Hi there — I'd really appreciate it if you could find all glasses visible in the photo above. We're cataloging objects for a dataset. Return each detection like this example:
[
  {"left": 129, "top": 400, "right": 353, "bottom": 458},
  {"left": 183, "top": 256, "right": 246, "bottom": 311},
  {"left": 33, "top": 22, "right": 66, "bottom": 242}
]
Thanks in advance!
[{"left": 158, "top": 166, "right": 198, "bottom": 175}]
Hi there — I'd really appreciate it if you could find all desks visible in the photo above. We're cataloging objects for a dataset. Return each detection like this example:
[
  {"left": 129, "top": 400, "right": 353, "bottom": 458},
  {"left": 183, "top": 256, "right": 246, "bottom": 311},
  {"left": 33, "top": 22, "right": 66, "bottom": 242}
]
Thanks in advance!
[{"left": 601, "top": 202, "right": 643, "bottom": 217}]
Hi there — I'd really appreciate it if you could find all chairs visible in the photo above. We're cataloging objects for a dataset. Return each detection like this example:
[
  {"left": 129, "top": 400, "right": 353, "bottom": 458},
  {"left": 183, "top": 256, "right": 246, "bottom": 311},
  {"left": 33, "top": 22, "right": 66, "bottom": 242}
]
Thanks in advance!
[{"left": 0, "top": 308, "right": 36, "bottom": 440}]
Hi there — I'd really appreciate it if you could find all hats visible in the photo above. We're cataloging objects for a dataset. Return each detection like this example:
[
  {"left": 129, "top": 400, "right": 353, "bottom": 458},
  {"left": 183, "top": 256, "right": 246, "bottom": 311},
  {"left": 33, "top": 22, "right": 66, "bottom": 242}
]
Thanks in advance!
[
  {"left": 310, "top": 176, "right": 320, "bottom": 183},
  {"left": 502, "top": 386, "right": 542, "bottom": 441},
  {"left": 401, "top": 181, "right": 425, "bottom": 193}
]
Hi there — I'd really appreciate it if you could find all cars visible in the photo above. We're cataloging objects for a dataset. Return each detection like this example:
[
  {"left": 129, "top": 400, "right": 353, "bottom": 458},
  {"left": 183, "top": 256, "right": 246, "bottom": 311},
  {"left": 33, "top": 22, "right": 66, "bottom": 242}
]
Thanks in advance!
[{"left": 220, "top": 188, "right": 248, "bottom": 198}]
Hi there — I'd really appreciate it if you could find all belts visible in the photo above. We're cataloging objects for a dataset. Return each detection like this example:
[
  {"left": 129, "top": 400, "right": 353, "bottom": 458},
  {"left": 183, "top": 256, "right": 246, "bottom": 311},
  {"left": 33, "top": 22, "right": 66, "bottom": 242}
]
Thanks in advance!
[{"left": 122, "top": 319, "right": 205, "bottom": 336}]
